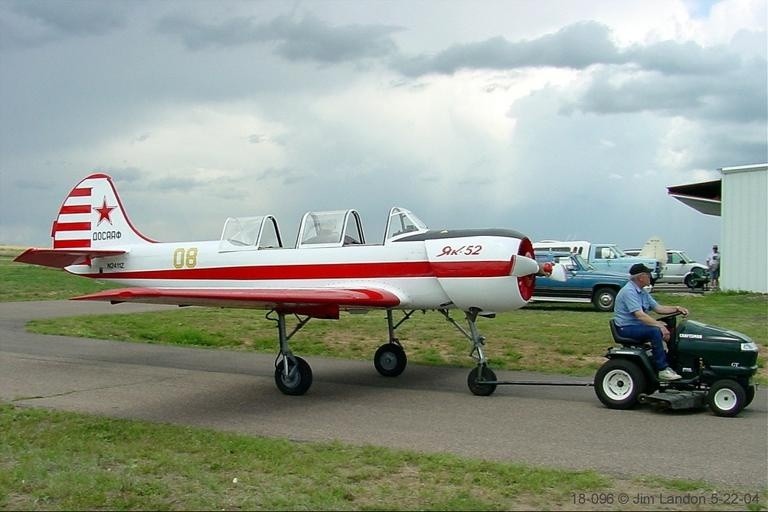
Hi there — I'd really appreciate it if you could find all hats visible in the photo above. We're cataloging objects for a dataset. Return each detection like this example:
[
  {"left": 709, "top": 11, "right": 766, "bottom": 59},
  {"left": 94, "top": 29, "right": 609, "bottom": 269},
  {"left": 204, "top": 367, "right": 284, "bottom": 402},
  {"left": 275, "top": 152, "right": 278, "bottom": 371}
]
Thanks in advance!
[{"left": 630, "top": 264, "right": 654, "bottom": 274}]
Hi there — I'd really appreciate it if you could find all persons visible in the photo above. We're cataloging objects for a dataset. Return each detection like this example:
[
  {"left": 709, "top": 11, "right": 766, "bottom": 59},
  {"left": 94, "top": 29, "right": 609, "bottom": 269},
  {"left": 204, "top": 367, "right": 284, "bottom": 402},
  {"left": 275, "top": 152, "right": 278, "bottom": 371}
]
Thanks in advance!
[
  {"left": 705, "top": 243, "right": 720, "bottom": 288},
  {"left": 613, "top": 263, "right": 689, "bottom": 381}
]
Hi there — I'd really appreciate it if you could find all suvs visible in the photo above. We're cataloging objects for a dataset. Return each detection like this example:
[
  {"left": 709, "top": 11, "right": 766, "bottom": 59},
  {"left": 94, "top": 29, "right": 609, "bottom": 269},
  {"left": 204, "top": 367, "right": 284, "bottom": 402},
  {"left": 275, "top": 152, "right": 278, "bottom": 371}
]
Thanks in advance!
[{"left": 533, "top": 253, "right": 630, "bottom": 311}]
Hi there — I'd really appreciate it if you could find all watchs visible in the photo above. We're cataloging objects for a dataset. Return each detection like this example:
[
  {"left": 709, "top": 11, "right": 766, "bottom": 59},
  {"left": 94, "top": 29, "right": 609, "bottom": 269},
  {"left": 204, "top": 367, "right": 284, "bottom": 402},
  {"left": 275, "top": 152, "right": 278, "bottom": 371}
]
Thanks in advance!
[{"left": 676, "top": 305, "right": 680, "bottom": 313}]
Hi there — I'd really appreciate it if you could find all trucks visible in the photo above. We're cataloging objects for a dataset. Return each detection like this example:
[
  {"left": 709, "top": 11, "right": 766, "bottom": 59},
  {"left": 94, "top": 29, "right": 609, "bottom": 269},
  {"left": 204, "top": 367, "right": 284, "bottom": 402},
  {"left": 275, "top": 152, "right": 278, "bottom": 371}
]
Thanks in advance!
[{"left": 534, "top": 239, "right": 662, "bottom": 284}]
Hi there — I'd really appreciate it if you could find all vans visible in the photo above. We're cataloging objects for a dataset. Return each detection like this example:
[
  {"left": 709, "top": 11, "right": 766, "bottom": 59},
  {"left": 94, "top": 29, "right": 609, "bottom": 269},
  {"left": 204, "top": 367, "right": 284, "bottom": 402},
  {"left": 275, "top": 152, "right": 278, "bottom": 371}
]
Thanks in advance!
[{"left": 626, "top": 250, "right": 710, "bottom": 290}]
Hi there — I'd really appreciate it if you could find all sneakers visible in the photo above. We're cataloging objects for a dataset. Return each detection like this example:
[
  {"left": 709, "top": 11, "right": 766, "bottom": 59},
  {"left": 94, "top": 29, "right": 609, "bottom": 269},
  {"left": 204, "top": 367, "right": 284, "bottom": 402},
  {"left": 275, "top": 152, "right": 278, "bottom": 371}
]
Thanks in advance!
[{"left": 658, "top": 367, "right": 682, "bottom": 381}]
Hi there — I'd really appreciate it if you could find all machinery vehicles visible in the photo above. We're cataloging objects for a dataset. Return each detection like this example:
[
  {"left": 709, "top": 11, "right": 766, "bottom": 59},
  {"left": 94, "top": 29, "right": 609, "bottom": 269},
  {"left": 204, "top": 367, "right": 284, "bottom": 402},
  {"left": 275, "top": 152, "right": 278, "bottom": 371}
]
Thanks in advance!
[{"left": 593, "top": 313, "right": 760, "bottom": 418}]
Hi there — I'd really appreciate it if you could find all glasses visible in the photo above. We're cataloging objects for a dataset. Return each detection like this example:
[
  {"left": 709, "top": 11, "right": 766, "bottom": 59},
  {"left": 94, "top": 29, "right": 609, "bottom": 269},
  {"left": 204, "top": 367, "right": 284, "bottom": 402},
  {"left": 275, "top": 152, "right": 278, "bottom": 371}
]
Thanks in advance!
[{"left": 713, "top": 247, "right": 717, "bottom": 250}]
